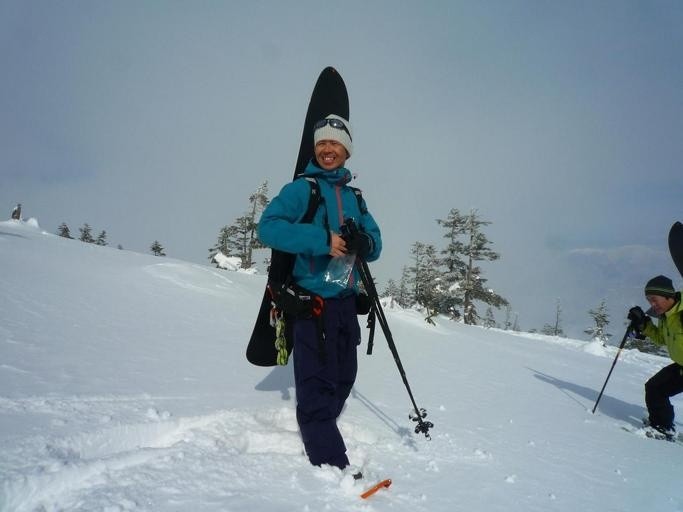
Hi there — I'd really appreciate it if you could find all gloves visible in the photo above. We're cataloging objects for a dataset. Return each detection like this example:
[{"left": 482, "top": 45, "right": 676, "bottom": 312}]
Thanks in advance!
[
  {"left": 339, "top": 217, "right": 365, "bottom": 255},
  {"left": 627, "top": 306, "right": 648, "bottom": 333}
]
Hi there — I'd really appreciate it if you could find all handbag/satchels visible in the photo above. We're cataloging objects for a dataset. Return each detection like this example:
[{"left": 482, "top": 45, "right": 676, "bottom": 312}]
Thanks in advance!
[{"left": 275, "top": 279, "right": 314, "bottom": 319}]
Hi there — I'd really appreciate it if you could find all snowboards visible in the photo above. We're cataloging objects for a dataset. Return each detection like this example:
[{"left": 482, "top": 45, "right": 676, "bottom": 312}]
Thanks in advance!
[
  {"left": 667, "top": 221, "right": 682, "bottom": 276},
  {"left": 246, "top": 66, "right": 348, "bottom": 367}
]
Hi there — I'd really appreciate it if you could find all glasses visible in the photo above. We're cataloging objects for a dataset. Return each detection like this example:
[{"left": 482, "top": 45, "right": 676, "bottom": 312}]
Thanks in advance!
[{"left": 316, "top": 119, "right": 346, "bottom": 131}]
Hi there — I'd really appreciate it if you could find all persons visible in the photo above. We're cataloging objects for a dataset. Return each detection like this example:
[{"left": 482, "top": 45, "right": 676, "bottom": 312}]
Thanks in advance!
[
  {"left": 626, "top": 274, "right": 683, "bottom": 442},
  {"left": 255, "top": 113, "right": 383, "bottom": 480}
]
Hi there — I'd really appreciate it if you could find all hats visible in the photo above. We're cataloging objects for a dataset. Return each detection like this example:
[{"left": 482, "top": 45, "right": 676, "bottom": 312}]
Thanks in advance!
[
  {"left": 645, "top": 275, "right": 677, "bottom": 298},
  {"left": 313, "top": 114, "right": 353, "bottom": 156}
]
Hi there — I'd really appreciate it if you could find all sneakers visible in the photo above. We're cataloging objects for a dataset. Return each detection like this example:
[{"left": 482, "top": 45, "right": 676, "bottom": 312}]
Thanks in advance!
[{"left": 645, "top": 423, "right": 677, "bottom": 442}]
